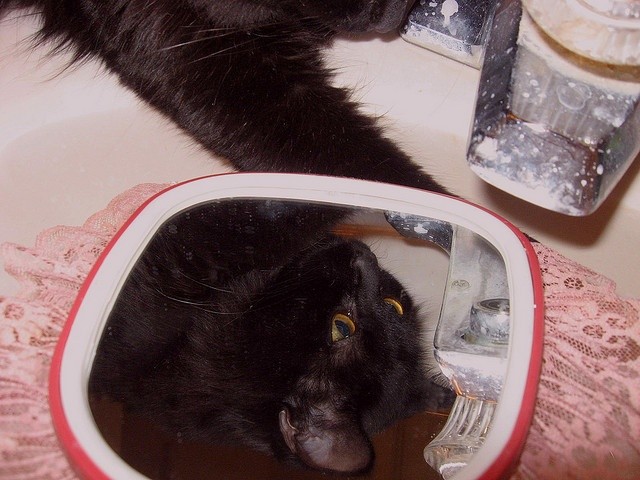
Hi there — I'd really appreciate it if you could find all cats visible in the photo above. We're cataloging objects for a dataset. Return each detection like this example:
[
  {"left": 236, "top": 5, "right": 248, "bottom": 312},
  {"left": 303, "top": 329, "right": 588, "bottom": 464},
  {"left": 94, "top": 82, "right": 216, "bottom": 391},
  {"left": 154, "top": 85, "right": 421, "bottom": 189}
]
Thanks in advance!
[
  {"left": 0, "top": 0, "right": 466, "bottom": 201},
  {"left": 87, "top": 198, "right": 442, "bottom": 479}
]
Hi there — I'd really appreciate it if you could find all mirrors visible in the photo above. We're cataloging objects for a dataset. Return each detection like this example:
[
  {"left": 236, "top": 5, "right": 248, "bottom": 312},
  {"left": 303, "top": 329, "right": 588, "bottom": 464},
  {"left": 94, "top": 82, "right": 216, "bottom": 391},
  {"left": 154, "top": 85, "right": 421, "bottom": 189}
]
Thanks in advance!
[{"left": 48, "top": 170, "right": 543, "bottom": 480}]
